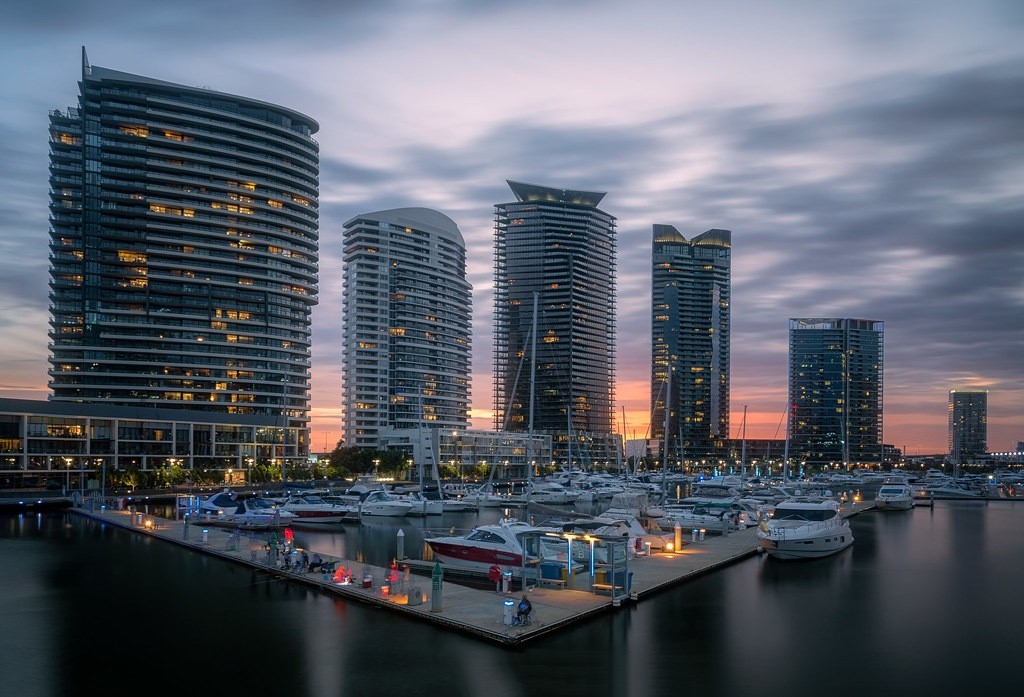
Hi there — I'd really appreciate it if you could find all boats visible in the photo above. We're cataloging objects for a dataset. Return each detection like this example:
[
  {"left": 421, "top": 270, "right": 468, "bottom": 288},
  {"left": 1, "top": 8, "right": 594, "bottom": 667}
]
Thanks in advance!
[
  {"left": 422, "top": 519, "right": 584, "bottom": 581},
  {"left": 875, "top": 483, "right": 915, "bottom": 513},
  {"left": 276, "top": 469, "right": 1024, "bottom": 584},
  {"left": 756, "top": 497, "right": 854, "bottom": 559},
  {"left": 192, "top": 491, "right": 300, "bottom": 530}
]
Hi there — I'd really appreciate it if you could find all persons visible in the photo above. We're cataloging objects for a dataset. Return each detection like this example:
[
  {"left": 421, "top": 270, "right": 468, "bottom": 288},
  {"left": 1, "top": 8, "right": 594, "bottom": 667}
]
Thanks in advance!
[
  {"left": 282, "top": 544, "right": 323, "bottom": 575},
  {"left": 388, "top": 562, "right": 399, "bottom": 595},
  {"left": 333, "top": 566, "right": 348, "bottom": 583},
  {"left": 401, "top": 563, "right": 411, "bottom": 596},
  {"left": 516, "top": 595, "right": 532, "bottom": 622}
]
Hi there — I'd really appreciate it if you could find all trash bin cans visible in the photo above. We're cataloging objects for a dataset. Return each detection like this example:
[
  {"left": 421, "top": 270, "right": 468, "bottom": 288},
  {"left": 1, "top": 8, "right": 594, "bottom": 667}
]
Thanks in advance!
[
  {"left": 594, "top": 568, "right": 610, "bottom": 592},
  {"left": 607, "top": 569, "right": 633, "bottom": 594},
  {"left": 562, "top": 568, "right": 575, "bottom": 587},
  {"left": 540, "top": 562, "right": 565, "bottom": 580}
]
